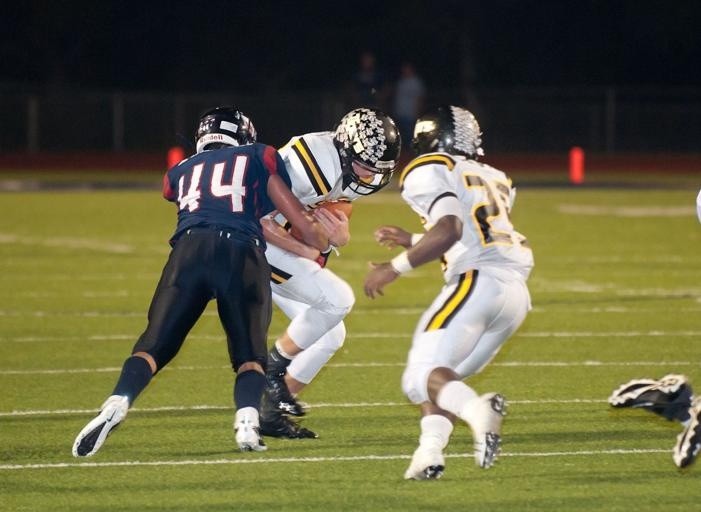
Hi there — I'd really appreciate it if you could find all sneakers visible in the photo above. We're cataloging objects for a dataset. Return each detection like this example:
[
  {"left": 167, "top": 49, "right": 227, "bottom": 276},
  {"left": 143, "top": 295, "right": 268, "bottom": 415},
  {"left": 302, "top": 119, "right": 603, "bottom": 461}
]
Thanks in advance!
[
  {"left": 403, "top": 446, "right": 444, "bottom": 480},
  {"left": 673, "top": 396, "right": 701, "bottom": 467},
  {"left": 470, "top": 392, "right": 508, "bottom": 470},
  {"left": 235, "top": 370, "right": 318, "bottom": 452},
  {"left": 70, "top": 395, "right": 130, "bottom": 458},
  {"left": 609, "top": 373, "right": 693, "bottom": 421}
]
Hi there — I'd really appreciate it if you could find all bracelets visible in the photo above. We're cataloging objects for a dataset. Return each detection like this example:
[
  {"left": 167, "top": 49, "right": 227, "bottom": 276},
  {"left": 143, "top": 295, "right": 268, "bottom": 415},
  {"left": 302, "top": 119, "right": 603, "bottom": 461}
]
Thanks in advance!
[
  {"left": 409, "top": 233, "right": 426, "bottom": 247},
  {"left": 321, "top": 246, "right": 333, "bottom": 266},
  {"left": 390, "top": 250, "right": 412, "bottom": 275}
]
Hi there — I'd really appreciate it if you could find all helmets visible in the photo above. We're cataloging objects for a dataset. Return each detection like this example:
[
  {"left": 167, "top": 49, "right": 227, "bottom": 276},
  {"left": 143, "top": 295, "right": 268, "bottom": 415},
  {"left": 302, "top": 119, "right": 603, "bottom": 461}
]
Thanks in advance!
[
  {"left": 334, "top": 105, "right": 403, "bottom": 195},
  {"left": 196, "top": 107, "right": 257, "bottom": 155},
  {"left": 412, "top": 103, "right": 484, "bottom": 160}
]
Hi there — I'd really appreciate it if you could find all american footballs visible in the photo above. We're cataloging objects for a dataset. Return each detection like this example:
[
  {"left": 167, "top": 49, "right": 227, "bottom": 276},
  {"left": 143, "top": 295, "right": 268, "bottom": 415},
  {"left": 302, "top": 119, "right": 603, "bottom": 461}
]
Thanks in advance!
[{"left": 290, "top": 198, "right": 352, "bottom": 241}]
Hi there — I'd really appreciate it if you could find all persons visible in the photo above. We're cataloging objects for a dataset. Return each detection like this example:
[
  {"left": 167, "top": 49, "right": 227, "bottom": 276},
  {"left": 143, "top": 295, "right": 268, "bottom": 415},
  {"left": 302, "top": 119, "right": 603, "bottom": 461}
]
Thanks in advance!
[
  {"left": 392, "top": 61, "right": 426, "bottom": 154},
  {"left": 364, "top": 103, "right": 537, "bottom": 483},
  {"left": 606, "top": 186, "right": 701, "bottom": 469},
  {"left": 69, "top": 103, "right": 336, "bottom": 457},
  {"left": 257, "top": 107, "right": 403, "bottom": 443}
]
